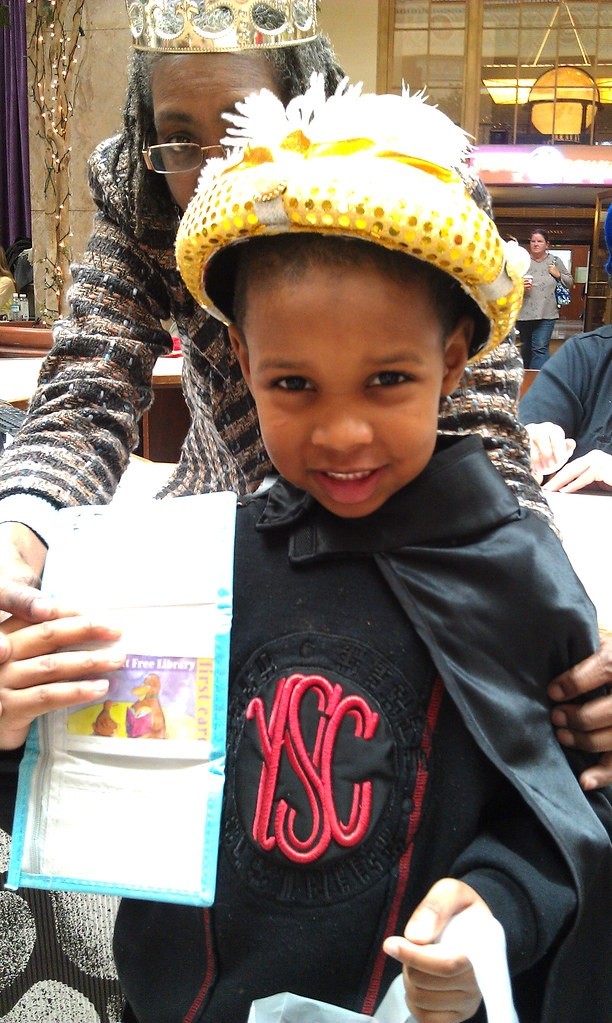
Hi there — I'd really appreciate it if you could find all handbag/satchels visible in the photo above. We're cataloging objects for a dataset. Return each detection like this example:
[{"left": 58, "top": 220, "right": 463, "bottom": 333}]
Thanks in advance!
[{"left": 552, "top": 257, "right": 571, "bottom": 307}]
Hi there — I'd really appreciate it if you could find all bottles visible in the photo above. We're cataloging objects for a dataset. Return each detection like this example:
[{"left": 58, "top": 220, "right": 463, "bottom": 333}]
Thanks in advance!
[
  {"left": 19, "top": 294, "right": 29, "bottom": 321},
  {"left": 11, "top": 293, "right": 22, "bottom": 321}
]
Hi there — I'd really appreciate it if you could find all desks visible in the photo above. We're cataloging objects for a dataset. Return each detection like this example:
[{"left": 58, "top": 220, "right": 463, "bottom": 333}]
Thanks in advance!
[{"left": 1, "top": 324, "right": 192, "bottom": 465}]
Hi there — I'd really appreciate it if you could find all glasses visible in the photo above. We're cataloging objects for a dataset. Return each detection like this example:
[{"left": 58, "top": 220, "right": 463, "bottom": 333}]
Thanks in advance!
[{"left": 142, "top": 133, "right": 236, "bottom": 174}]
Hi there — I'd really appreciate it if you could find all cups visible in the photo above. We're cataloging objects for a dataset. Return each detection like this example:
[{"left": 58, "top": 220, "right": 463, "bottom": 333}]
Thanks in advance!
[{"left": 524, "top": 275, "right": 533, "bottom": 289}]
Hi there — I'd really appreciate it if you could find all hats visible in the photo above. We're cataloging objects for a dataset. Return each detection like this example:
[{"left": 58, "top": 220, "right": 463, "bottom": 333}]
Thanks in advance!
[
  {"left": 604, "top": 206, "right": 612, "bottom": 274},
  {"left": 174, "top": 71, "right": 532, "bottom": 363}
]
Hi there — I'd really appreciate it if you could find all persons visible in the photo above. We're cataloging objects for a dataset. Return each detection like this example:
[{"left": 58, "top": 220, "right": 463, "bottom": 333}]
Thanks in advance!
[
  {"left": 514, "top": 229, "right": 574, "bottom": 369},
  {"left": 0, "top": 0, "right": 612, "bottom": 795},
  {"left": 518, "top": 205, "right": 612, "bottom": 496},
  {"left": 0, "top": 93, "right": 612, "bottom": 1023},
  {"left": 0, "top": 244, "right": 18, "bottom": 320}
]
